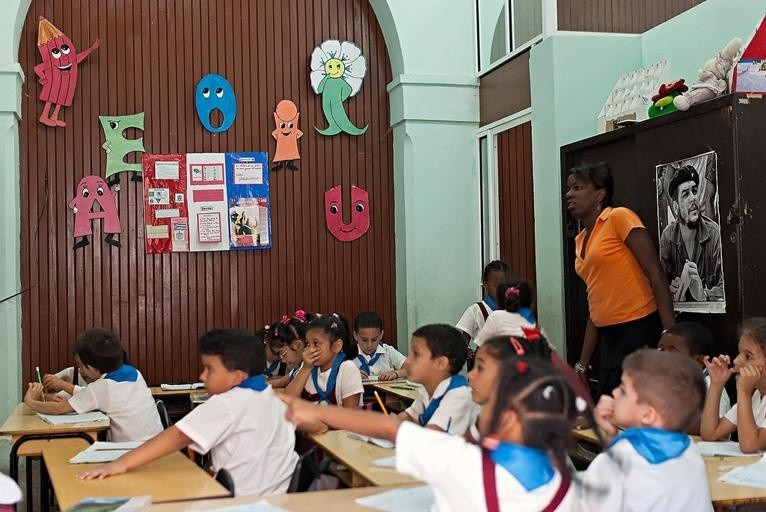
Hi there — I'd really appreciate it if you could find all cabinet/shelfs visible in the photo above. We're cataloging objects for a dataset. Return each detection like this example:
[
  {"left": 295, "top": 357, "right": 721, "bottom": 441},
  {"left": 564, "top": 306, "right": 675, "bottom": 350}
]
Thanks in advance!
[{"left": 557, "top": 88, "right": 766, "bottom": 443}]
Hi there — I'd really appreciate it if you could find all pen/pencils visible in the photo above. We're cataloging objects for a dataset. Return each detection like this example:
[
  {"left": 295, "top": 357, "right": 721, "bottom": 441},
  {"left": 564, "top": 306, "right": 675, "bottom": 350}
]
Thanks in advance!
[
  {"left": 36, "top": 366, "right": 46, "bottom": 402},
  {"left": 374, "top": 391, "right": 388, "bottom": 416},
  {"left": 95, "top": 448, "right": 134, "bottom": 451},
  {"left": 446, "top": 417, "right": 452, "bottom": 432},
  {"left": 389, "top": 385, "right": 415, "bottom": 391}
]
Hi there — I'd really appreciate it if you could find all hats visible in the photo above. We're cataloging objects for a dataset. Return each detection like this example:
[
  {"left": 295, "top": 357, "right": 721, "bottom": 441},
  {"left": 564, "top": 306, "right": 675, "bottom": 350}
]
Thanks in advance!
[{"left": 668, "top": 165, "right": 699, "bottom": 196}]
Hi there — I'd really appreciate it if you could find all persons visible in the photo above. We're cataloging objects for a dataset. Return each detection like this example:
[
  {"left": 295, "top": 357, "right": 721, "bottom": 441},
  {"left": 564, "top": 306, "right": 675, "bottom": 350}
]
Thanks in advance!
[
  {"left": 564, "top": 160, "right": 678, "bottom": 394},
  {"left": 659, "top": 154, "right": 725, "bottom": 303},
  {"left": 23, "top": 260, "right": 766, "bottom": 511}
]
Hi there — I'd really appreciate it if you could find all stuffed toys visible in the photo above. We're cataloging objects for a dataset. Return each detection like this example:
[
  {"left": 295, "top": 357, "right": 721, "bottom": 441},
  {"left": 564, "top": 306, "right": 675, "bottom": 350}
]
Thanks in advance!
[
  {"left": 648, "top": 78, "right": 688, "bottom": 119},
  {"left": 672, "top": 37, "right": 743, "bottom": 112}
]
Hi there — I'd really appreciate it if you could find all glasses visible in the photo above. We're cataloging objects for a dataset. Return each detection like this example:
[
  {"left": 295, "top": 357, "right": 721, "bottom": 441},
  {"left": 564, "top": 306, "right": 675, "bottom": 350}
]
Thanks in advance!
[{"left": 277, "top": 344, "right": 294, "bottom": 360}]
